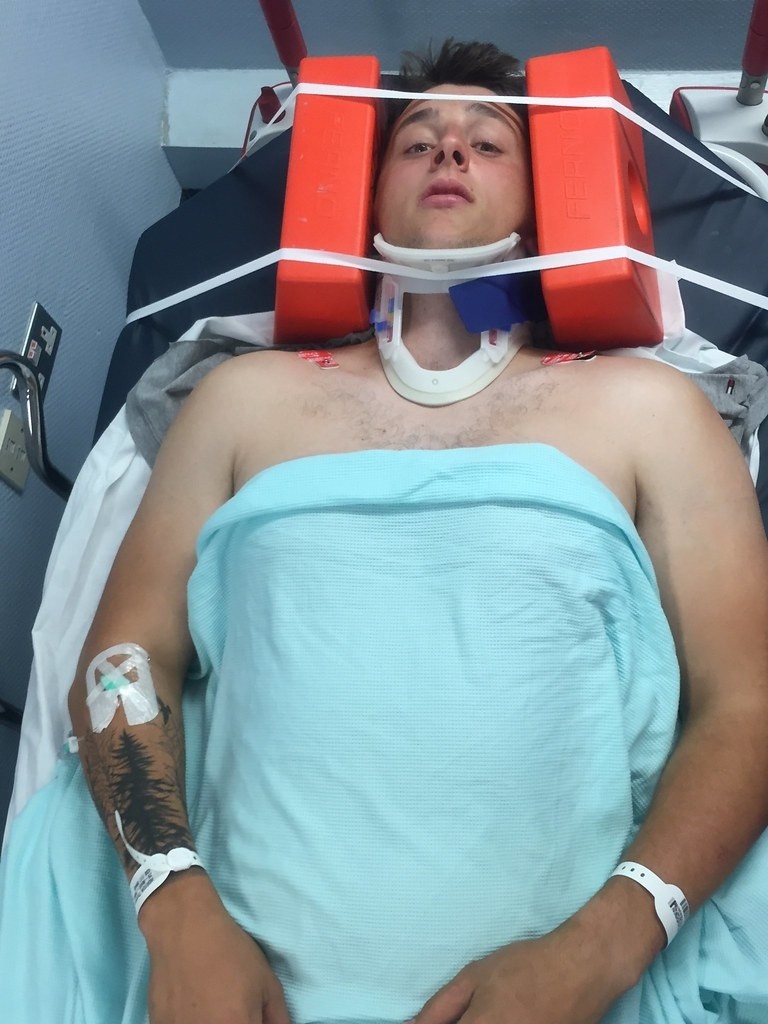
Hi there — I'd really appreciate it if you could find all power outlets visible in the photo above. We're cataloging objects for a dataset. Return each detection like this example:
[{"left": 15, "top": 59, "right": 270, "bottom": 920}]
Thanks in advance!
[{"left": 11, "top": 301, "right": 62, "bottom": 407}]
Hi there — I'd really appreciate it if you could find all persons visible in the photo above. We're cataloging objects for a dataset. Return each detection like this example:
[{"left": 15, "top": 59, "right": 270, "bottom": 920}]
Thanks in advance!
[{"left": 64, "top": 32, "right": 768, "bottom": 1024}]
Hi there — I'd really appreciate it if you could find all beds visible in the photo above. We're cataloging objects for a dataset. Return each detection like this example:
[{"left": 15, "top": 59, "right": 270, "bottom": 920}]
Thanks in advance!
[{"left": 1, "top": 67, "right": 768, "bottom": 1024}]
